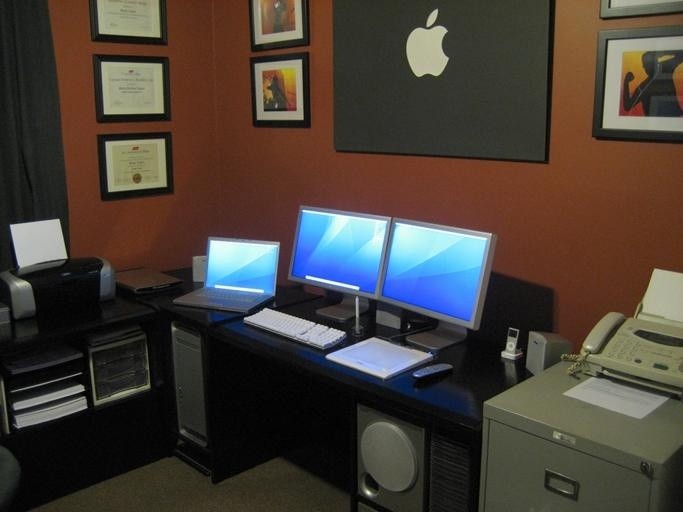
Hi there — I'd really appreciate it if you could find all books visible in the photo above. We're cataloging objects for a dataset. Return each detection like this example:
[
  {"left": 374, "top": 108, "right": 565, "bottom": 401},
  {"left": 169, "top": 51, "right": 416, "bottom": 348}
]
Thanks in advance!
[
  {"left": 11, "top": 394, "right": 88, "bottom": 429},
  {"left": 114, "top": 268, "right": 184, "bottom": 294},
  {"left": 324, "top": 335, "right": 435, "bottom": 382},
  {"left": 7, "top": 368, "right": 86, "bottom": 413}
]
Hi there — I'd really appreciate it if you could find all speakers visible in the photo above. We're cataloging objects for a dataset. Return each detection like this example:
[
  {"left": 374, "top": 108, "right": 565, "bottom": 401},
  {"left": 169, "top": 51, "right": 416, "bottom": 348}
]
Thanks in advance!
[{"left": 356, "top": 404, "right": 426, "bottom": 512}]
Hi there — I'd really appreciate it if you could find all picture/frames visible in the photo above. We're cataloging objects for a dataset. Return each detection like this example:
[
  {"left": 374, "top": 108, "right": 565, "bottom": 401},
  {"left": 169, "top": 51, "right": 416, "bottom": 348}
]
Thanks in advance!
[
  {"left": 92, "top": 53, "right": 171, "bottom": 123},
  {"left": 95, "top": 130, "right": 173, "bottom": 202},
  {"left": 249, "top": 1, "right": 312, "bottom": 53},
  {"left": 248, "top": 52, "right": 311, "bottom": 130},
  {"left": 597, "top": 0, "right": 682, "bottom": 20},
  {"left": 87, "top": 0, "right": 169, "bottom": 45},
  {"left": 588, "top": 24, "right": 682, "bottom": 145}
]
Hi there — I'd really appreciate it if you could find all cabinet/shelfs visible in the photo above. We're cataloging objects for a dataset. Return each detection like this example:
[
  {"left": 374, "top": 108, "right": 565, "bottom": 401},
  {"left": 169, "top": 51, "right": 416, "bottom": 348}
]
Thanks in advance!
[
  {"left": 482, "top": 350, "right": 682, "bottom": 511},
  {"left": 0, "top": 297, "right": 171, "bottom": 511}
]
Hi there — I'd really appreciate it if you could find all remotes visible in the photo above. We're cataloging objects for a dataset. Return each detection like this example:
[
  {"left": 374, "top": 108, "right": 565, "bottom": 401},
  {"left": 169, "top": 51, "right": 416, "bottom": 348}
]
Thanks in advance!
[{"left": 412, "top": 363, "right": 454, "bottom": 377}]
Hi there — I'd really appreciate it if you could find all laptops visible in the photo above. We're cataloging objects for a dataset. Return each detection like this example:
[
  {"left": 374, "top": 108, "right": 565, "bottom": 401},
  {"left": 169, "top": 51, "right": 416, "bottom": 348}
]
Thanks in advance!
[{"left": 173, "top": 237, "right": 280, "bottom": 313}]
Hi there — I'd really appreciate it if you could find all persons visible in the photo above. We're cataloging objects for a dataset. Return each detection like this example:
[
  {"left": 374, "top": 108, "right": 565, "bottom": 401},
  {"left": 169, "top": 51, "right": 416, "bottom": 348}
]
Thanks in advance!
[
  {"left": 265, "top": 73, "right": 288, "bottom": 110},
  {"left": 622, "top": 48, "right": 683, "bottom": 117}
]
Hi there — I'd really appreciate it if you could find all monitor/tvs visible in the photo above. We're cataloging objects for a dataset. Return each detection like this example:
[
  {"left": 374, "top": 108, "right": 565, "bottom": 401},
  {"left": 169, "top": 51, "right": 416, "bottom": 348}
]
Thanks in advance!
[
  {"left": 287, "top": 205, "right": 392, "bottom": 323},
  {"left": 377, "top": 218, "right": 497, "bottom": 350}
]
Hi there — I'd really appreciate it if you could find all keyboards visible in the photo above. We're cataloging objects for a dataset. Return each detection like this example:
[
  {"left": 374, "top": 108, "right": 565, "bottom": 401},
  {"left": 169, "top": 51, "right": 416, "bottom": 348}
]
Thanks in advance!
[{"left": 244, "top": 308, "right": 348, "bottom": 350}]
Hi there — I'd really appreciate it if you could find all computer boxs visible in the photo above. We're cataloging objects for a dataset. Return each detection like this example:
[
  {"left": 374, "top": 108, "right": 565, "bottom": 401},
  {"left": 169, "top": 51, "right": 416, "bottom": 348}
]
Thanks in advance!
[{"left": 170, "top": 322, "right": 207, "bottom": 448}]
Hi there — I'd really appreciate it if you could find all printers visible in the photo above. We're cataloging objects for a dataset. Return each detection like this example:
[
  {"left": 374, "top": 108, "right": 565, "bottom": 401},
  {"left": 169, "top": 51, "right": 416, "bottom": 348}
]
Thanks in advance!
[{"left": 0, "top": 256, "right": 116, "bottom": 319}]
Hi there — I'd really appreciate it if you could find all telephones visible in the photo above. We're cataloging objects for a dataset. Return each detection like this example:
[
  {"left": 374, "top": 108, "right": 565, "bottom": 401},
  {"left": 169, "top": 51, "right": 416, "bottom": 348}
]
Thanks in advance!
[{"left": 580, "top": 302, "right": 683, "bottom": 397}]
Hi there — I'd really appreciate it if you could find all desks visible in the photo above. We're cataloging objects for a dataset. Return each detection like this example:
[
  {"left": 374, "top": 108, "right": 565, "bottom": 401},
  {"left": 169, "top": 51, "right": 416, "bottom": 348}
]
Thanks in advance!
[
  {"left": 216, "top": 293, "right": 554, "bottom": 511},
  {"left": 112, "top": 264, "right": 326, "bottom": 334}
]
